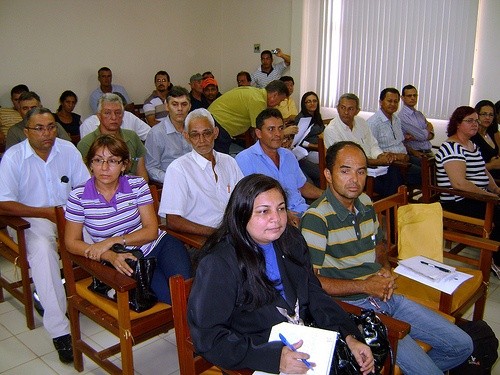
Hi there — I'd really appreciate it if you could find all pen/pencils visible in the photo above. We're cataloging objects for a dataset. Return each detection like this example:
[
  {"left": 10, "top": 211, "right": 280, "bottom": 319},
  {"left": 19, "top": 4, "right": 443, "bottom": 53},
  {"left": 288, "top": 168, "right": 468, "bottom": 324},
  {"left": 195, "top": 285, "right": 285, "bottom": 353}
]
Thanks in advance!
[
  {"left": 421, "top": 261, "right": 451, "bottom": 273},
  {"left": 278, "top": 333, "right": 315, "bottom": 371}
]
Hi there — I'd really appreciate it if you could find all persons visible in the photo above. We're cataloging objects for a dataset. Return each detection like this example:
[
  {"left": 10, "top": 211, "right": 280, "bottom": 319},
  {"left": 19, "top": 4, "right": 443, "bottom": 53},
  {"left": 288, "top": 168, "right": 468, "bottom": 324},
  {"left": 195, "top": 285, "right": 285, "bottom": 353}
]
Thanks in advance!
[
  {"left": 187, "top": 173, "right": 375, "bottom": 375},
  {"left": 77, "top": 93, "right": 148, "bottom": 185},
  {"left": 397, "top": 84, "right": 437, "bottom": 186},
  {"left": 188, "top": 48, "right": 328, "bottom": 188},
  {"left": 64, "top": 134, "right": 195, "bottom": 306},
  {"left": 469, "top": 100, "right": 500, "bottom": 189},
  {"left": 299, "top": 141, "right": 474, "bottom": 375},
  {"left": 144, "top": 86, "right": 192, "bottom": 184},
  {"left": 0, "top": 67, "right": 171, "bottom": 161},
  {"left": 158, "top": 108, "right": 245, "bottom": 236},
  {"left": 235, "top": 108, "right": 326, "bottom": 229},
  {"left": 435, "top": 106, "right": 500, "bottom": 280},
  {"left": 365, "top": 87, "right": 430, "bottom": 204},
  {"left": 323, "top": 92, "right": 407, "bottom": 247},
  {"left": 0, "top": 107, "right": 95, "bottom": 364}
]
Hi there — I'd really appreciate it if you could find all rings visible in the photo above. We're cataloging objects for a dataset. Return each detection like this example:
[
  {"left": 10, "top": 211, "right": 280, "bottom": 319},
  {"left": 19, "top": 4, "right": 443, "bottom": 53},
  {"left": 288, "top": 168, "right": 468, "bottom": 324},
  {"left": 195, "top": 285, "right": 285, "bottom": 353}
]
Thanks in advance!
[
  {"left": 93, "top": 256, "right": 96, "bottom": 257},
  {"left": 118, "top": 264, "right": 122, "bottom": 267}
]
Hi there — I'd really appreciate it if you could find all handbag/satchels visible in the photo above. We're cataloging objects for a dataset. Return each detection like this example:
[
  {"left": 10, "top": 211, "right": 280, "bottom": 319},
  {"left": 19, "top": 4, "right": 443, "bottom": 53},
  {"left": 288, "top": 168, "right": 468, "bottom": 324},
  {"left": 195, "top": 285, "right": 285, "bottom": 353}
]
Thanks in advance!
[
  {"left": 87, "top": 243, "right": 160, "bottom": 313},
  {"left": 442, "top": 319, "right": 499, "bottom": 375},
  {"left": 333, "top": 309, "right": 388, "bottom": 375}
]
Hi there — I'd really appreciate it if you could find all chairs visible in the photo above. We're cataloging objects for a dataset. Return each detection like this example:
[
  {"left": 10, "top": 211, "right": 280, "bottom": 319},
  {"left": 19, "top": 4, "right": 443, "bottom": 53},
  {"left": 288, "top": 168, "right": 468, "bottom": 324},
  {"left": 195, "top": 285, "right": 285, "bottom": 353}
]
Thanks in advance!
[{"left": 0, "top": 103, "right": 500, "bottom": 375}]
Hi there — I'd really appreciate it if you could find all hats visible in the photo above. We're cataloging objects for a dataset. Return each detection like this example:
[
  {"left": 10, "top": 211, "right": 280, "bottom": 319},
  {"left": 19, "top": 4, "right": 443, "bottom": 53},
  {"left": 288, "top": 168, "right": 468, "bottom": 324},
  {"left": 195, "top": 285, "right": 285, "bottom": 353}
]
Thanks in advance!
[
  {"left": 190, "top": 73, "right": 203, "bottom": 84},
  {"left": 202, "top": 78, "right": 218, "bottom": 93}
]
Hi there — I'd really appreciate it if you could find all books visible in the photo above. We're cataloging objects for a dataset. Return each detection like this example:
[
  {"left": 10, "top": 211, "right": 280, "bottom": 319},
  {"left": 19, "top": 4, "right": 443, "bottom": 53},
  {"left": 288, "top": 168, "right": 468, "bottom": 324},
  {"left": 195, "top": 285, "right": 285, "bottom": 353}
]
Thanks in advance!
[
  {"left": 366, "top": 166, "right": 389, "bottom": 177},
  {"left": 253, "top": 322, "right": 338, "bottom": 375},
  {"left": 397, "top": 255, "right": 456, "bottom": 283}
]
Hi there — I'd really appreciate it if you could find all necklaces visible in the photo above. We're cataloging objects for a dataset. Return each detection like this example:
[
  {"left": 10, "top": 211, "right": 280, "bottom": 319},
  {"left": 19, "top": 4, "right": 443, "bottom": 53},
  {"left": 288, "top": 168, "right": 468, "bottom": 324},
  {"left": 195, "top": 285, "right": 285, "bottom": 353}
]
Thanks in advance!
[{"left": 479, "top": 131, "right": 489, "bottom": 139}]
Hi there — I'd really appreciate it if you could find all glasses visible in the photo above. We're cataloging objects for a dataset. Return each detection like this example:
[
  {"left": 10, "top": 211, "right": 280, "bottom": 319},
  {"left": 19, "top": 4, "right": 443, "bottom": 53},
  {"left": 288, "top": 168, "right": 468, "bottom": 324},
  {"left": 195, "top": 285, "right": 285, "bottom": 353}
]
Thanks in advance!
[
  {"left": 188, "top": 131, "right": 214, "bottom": 138},
  {"left": 405, "top": 94, "right": 417, "bottom": 97},
  {"left": 478, "top": 112, "right": 494, "bottom": 117},
  {"left": 157, "top": 79, "right": 166, "bottom": 82},
  {"left": 305, "top": 100, "right": 318, "bottom": 103},
  {"left": 341, "top": 105, "right": 356, "bottom": 111},
  {"left": 90, "top": 159, "right": 123, "bottom": 166},
  {"left": 462, "top": 119, "right": 479, "bottom": 123},
  {"left": 25, "top": 126, "right": 58, "bottom": 132}
]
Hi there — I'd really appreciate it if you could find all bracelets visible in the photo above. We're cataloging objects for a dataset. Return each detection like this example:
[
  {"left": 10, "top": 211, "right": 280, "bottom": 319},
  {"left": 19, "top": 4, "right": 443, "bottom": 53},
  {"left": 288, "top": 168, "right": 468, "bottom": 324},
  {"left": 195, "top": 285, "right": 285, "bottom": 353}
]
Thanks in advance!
[
  {"left": 122, "top": 235, "right": 126, "bottom": 247},
  {"left": 493, "top": 187, "right": 498, "bottom": 192}
]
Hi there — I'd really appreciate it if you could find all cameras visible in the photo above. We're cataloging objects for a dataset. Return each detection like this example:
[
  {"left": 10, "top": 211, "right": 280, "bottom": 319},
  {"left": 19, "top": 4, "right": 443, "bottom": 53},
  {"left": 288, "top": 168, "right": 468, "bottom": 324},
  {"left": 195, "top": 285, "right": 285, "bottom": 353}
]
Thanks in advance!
[{"left": 271, "top": 50, "right": 278, "bottom": 54}]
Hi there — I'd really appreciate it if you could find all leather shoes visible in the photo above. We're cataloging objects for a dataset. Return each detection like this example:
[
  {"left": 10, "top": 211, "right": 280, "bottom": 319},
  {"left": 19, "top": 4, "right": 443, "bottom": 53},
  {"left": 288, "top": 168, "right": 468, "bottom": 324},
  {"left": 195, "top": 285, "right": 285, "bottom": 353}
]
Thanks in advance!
[{"left": 53, "top": 334, "right": 74, "bottom": 364}]
